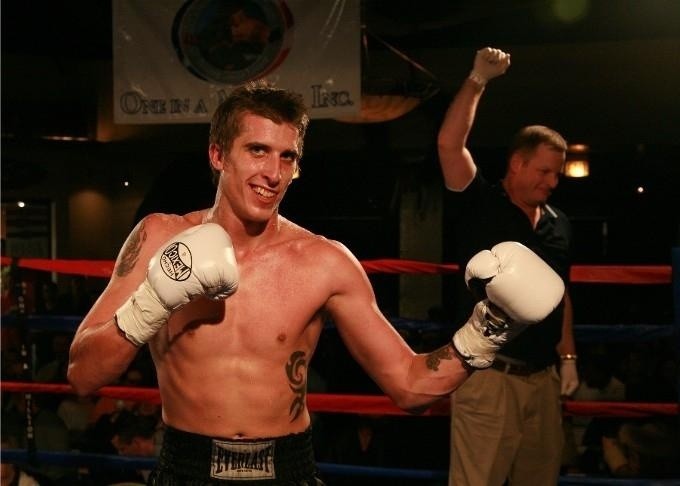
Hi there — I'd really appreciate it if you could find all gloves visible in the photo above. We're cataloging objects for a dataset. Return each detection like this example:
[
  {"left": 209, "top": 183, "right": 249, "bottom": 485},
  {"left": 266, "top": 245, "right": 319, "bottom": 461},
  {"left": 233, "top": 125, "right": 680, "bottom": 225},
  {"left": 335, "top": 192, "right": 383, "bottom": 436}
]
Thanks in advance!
[
  {"left": 114, "top": 222, "right": 239, "bottom": 348},
  {"left": 453, "top": 241, "right": 567, "bottom": 369}
]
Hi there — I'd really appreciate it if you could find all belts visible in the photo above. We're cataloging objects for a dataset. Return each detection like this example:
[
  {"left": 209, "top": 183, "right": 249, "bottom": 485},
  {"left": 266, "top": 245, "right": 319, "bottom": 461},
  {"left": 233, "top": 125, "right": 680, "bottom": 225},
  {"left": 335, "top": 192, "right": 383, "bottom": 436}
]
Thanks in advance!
[{"left": 493, "top": 358, "right": 545, "bottom": 376}]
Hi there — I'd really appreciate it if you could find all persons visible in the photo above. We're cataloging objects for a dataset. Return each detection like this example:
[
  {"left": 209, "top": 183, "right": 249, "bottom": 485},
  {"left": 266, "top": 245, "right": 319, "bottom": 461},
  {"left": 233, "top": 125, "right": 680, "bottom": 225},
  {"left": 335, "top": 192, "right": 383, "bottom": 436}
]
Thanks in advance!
[
  {"left": 325, "top": 379, "right": 394, "bottom": 466},
  {"left": 1, "top": 329, "right": 164, "bottom": 486},
  {"left": 66, "top": 79, "right": 565, "bottom": 486},
  {"left": 578, "top": 349, "right": 662, "bottom": 476},
  {"left": 436, "top": 46, "right": 580, "bottom": 486}
]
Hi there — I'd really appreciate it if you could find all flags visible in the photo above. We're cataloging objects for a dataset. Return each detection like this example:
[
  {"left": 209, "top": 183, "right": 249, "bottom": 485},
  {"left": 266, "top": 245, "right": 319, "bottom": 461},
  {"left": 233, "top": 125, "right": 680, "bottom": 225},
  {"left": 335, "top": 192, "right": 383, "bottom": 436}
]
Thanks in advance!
[{"left": 111, "top": 0, "right": 362, "bottom": 125}]
